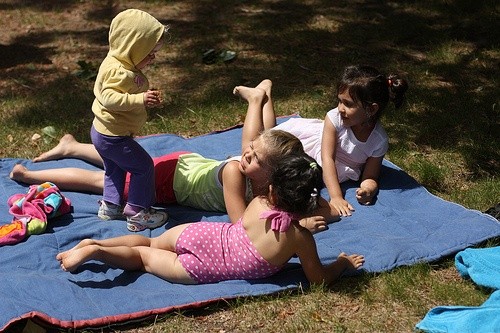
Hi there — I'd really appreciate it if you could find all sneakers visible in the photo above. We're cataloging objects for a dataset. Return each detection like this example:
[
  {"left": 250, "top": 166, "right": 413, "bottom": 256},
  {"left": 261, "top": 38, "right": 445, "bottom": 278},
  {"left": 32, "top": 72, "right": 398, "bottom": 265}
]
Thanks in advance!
[
  {"left": 97, "top": 199, "right": 124, "bottom": 221},
  {"left": 126, "top": 207, "right": 169, "bottom": 233}
]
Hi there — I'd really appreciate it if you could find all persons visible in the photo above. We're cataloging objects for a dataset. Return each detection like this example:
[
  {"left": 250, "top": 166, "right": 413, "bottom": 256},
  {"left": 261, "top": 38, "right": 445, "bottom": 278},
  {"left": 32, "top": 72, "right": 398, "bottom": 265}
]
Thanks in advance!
[
  {"left": 56, "top": 157, "right": 364, "bottom": 289},
  {"left": 232, "top": 64, "right": 404, "bottom": 218},
  {"left": 91, "top": 9, "right": 174, "bottom": 231},
  {"left": 9, "top": 129, "right": 341, "bottom": 235}
]
